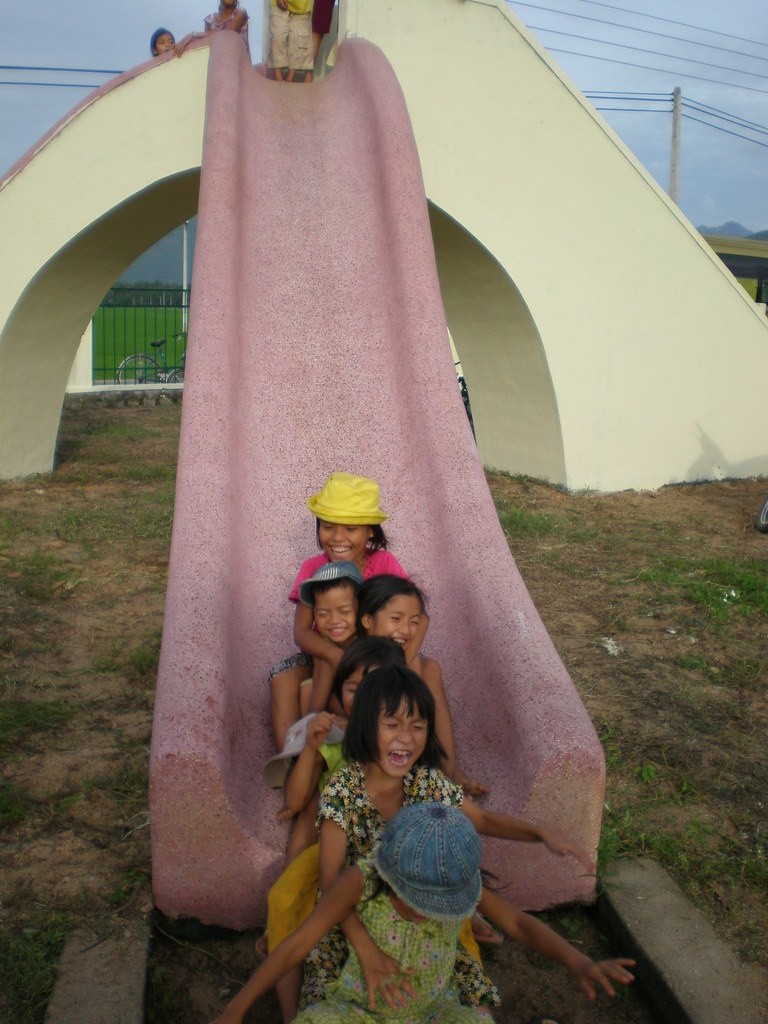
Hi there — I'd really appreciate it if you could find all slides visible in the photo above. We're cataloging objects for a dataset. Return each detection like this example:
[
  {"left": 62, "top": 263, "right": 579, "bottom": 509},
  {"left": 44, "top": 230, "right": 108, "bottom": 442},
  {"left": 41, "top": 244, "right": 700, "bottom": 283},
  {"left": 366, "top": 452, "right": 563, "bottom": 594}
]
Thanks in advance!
[{"left": 148, "top": 32, "right": 603, "bottom": 927}]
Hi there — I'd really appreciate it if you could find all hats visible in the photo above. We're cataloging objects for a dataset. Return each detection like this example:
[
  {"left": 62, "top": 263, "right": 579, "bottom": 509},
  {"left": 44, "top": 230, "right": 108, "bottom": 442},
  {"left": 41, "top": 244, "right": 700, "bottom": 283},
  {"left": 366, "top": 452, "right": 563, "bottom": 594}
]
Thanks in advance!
[
  {"left": 374, "top": 802, "right": 483, "bottom": 919},
  {"left": 306, "top": 472, "right": 389, "bottom": 525},
  {"left": 298, "top": 560, "right": 364, "bottom": 607},
  {"left": 261, "top": 713, "right": 345, "bottom": 790}
]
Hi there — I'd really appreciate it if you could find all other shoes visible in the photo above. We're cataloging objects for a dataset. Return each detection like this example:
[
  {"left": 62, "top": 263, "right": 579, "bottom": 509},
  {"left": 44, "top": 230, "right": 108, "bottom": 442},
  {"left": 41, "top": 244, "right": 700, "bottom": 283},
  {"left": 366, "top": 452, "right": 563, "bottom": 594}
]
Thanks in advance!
[
  {"left": 470, "top": 913, "right": 504, "bottom": 944},
  {"left": 256, "top": 937, "right": 268, "bottom": 956}
]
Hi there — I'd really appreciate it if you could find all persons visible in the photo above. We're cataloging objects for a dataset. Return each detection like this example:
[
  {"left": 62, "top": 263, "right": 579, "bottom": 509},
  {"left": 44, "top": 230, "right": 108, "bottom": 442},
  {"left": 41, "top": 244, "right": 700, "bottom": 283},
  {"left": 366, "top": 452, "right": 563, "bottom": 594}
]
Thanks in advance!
[
  {"left": 270, "top": 472, "right": 511, "bottom": 890},
  {"left": 150, "top": 27, "right": 175, "bottom": 56},
  {"left": 264, "top": 0, "right": 336, "bottom": 83},
  {"left": 253, "top": 636, "right": 505, "bottom": 960},
  {"left": 265, "top": 666, "right": 596, "bottom": 1024},
  {"left": 211, "top": 801, "right": 638, "bottom": 1024},
  {"left": 175, "top": 0, "right": 252, "bottom": 65}
]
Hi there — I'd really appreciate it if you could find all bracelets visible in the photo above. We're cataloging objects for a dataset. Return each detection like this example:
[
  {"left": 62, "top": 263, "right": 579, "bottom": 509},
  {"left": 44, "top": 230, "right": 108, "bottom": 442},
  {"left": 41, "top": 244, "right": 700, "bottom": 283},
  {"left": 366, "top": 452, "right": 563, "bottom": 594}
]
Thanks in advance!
[{"left": 193, "top": 31, "right": 197, "bottom": 38}]
